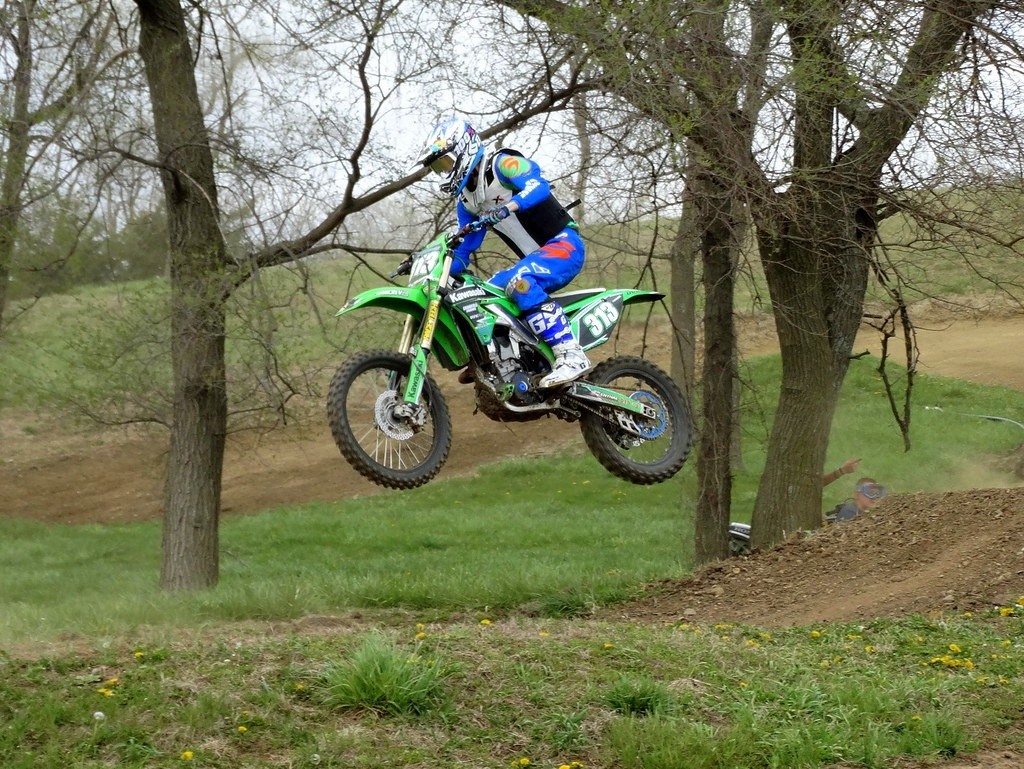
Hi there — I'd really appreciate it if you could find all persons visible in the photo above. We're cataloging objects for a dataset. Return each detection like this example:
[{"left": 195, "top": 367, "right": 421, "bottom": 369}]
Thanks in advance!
[{"left": 401, "top": 120, "right": 592, "bottom": 388}]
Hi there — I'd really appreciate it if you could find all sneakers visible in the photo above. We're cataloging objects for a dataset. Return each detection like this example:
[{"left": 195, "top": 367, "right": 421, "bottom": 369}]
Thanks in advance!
[
  {"left": 459, "top": 368, "right": 475, "bottom": 384},
  {"left": 539, "top": 346, "right": 593, "bottom": 388}
]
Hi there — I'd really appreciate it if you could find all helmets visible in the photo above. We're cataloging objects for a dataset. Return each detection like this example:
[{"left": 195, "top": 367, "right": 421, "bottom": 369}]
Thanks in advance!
[{"left": 415, "top": 119, "right": 484, "bottom": 199}]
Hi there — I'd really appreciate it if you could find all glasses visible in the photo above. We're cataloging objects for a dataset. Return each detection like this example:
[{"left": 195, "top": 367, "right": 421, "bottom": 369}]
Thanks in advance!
[{"left": 430, "top": 125, "right": 475, "bottom": 181}]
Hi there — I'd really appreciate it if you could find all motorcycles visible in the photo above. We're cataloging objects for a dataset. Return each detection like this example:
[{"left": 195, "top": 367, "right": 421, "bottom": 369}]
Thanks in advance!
[{"left": 327, "top": 209, "right": 694, "bottom": 489}]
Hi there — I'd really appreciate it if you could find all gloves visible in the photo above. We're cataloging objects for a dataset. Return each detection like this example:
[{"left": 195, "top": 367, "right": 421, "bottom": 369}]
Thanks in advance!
[
  {"left": 479, "top": 204, "right": 511, "bottom": 228},
  {"left": 396, "top": 254, "right": 414, "bottom": 276}
]
